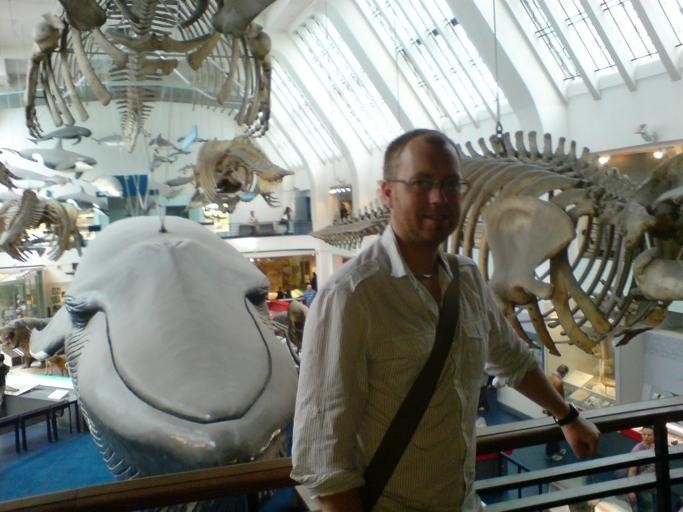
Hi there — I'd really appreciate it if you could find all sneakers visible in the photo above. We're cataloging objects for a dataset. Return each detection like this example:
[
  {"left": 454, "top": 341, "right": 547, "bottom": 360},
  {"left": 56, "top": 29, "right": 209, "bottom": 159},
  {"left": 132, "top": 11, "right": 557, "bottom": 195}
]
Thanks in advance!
[{"left": 544, "top": 448, "right": 567, "bottom": 461}]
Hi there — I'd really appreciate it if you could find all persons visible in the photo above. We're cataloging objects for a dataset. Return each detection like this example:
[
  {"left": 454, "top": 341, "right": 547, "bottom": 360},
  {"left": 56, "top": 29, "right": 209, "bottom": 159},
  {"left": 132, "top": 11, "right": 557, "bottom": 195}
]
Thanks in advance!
[
  {"left": 279, "top": 206, "right": 292, "bottom": 234},
  {"left": 310, "top": 272, "right": 316, "bottom": 290},
  {"left": 340, "top": 202, "right": 347, "bottom": 218},
  {"left": 286, "top": 128, "right": 603, "bottom": 512},
  {"left": 303, "top": 283, "right": 317, "bottom": 308},
  {"left": 541, "top": 363, "right": 569, "bottom": 461},
  {"left": 247, "top": 210, "right": 257, "bottom": 236},
  {"left": 283, "top": 289, "right": 291, "bottom": 298},
  {"left": 0, "top": 353, "right": 10, "bottom": 407},
  {"left": 276, "top": 286, "right": 283, "bottom": 299},
  {"left": 624, "top": 424, "right": 679, "bottom": 512}
]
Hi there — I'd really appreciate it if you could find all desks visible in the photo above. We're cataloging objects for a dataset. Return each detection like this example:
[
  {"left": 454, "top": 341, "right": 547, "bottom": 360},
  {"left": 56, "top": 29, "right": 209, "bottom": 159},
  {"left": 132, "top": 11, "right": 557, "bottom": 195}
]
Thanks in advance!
[{"left": 0, "top": 370, "right": 80, "bottom": 455}]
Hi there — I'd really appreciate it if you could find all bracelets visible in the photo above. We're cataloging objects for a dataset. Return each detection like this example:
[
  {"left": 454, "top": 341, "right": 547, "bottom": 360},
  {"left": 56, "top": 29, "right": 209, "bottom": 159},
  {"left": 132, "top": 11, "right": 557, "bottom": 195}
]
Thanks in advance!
[{"left": 552, "top": 402, "right": 579, "bottom": 427}]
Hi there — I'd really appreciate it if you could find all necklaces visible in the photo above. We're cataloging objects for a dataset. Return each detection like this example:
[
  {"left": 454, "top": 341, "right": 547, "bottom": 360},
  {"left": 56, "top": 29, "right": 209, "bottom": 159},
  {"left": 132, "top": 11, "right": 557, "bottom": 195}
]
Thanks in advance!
[{"left": 410, "top": 268, "right": 438, "bottom": 282}]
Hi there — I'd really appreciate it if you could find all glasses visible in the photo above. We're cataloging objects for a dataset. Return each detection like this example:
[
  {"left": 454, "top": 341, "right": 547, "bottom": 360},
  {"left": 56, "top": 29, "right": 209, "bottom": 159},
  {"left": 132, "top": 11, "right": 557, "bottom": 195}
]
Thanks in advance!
[{"left": 384, "top": 175, "right": 470, "bottom": 196}]
